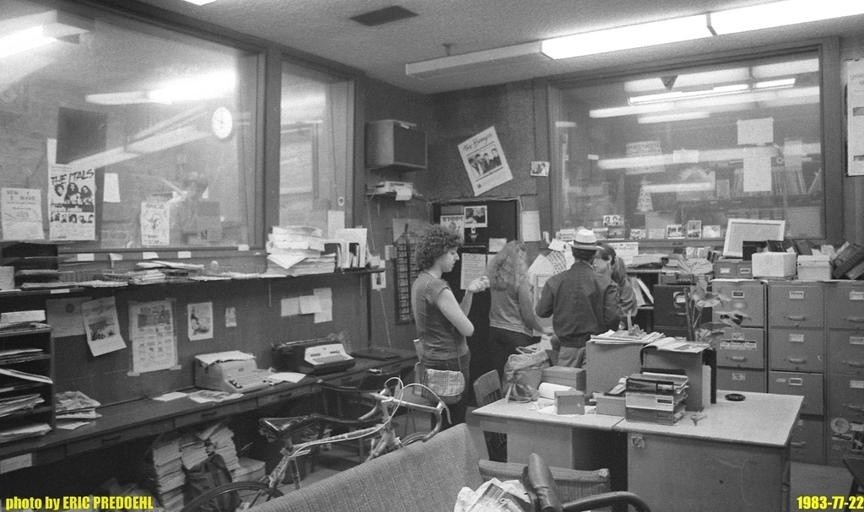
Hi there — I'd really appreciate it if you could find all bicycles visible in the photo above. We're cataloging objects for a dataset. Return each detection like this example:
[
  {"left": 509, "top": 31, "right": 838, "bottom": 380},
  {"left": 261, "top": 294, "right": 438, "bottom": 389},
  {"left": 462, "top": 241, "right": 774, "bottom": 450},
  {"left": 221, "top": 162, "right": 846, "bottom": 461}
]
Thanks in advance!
[{"left": 175, "top": 375, "right": 454, "bottom": 512}]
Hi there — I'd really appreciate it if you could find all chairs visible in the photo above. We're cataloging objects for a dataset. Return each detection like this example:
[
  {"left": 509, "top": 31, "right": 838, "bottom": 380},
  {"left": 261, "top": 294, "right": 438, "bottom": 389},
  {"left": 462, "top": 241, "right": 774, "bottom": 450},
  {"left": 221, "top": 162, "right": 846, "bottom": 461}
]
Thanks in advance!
[
  {"left": 522, "top": 450, "right": 652, "bottom": 511},
  {"left": 471, "top": 368, "right": 508, "bottom": 462}
]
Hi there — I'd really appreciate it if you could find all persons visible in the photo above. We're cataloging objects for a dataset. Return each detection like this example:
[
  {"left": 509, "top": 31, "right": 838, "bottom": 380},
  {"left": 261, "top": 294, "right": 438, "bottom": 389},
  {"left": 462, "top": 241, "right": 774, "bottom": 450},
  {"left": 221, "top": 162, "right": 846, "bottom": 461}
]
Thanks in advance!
[
  {"left": 78, "top": 185, "right": 94, "bottom": 206},
  {"left": 593, "top": 245, "right": 637, "bottom": 328},
  {"left": 469, "top": 148, "right": 502, "bottom": 179},
  {"left": 51, "top": 184, "right": 65, "bottom": 204},
  {"left": 485, "top": 240, "right": 543, "bottom": 362},
  {"left": 167, "top": 173, "right": 209, "bottom": 242},
  {"left": 410, "top": 224, "right": 489, "bottom": 433},
  {"left": 534, "top": 229, "right": 621, "bottom": 368},
  {"left": 64, "top": 183, "right": 82, "bottom": 205}
]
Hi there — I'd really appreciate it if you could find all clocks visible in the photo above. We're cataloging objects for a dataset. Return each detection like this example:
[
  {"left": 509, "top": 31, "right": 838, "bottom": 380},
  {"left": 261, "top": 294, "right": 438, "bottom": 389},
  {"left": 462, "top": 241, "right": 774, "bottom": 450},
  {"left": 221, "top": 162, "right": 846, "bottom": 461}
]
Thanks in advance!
[{"left": 209, "top": 104, "right": 235, "bottom": 140}]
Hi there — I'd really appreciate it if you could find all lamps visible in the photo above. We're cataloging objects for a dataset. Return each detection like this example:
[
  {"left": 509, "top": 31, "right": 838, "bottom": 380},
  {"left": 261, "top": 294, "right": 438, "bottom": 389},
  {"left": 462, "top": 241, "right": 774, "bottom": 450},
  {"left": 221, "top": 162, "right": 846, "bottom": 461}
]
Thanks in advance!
[{"left": 407, "top": 1, "right": 864, "bottom": 93}]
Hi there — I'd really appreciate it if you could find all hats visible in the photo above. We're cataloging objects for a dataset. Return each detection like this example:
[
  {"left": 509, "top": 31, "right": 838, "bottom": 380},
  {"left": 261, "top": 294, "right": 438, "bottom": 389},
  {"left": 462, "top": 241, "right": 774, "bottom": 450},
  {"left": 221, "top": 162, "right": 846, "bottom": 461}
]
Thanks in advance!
[{"left": 567, "top": 230, "right": 605, "bottom": 251}]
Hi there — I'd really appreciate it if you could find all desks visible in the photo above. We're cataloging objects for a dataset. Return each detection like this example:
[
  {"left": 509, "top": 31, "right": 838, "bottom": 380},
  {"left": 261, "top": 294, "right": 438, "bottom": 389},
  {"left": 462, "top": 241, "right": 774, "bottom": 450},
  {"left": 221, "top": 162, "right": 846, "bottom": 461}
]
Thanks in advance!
[
  {"left": 613, "top": 383, "right": 806, "bottom": 510},
  {"left": 471, "top": 386, "right": 635, "bottom": 469},
  {"left": 0, "top": 341, "right": 417, "bottom": 511}
]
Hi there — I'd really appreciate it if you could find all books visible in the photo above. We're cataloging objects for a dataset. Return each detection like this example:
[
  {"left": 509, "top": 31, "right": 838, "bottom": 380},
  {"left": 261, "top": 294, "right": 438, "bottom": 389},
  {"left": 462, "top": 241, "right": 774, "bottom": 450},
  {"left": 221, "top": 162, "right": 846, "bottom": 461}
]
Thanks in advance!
[{"left": 137, "top": 421, "right": 241, "bottom": 512}]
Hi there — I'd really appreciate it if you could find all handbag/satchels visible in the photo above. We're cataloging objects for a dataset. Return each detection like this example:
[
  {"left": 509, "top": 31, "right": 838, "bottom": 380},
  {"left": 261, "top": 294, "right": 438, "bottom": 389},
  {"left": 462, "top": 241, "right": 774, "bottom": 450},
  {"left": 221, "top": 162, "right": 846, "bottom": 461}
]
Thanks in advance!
[{"left": 421, "top": 368, "right": 465, "bottom": 406}]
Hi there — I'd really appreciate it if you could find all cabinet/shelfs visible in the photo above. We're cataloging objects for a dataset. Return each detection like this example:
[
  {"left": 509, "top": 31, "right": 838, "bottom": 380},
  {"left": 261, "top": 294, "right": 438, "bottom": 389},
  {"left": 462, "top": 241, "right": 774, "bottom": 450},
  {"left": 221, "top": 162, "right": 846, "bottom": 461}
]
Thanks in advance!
[{"left": 709, "top": 278, "right": 862, "bottom": 471}]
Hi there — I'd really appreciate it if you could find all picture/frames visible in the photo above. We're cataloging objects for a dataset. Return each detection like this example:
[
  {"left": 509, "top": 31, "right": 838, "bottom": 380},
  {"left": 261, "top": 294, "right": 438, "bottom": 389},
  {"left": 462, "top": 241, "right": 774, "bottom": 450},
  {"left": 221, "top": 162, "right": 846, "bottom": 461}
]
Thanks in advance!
[{"left": 721, "top": 217, "right": 786, "bottom": 259}]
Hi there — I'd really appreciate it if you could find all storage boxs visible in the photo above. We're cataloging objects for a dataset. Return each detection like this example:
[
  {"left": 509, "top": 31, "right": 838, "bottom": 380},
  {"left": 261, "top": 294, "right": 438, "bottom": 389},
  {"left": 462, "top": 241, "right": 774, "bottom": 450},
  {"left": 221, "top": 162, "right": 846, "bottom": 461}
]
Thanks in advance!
[
  {"left": 797, "top": 255, "right": 834, "bottom": 279},
  {"left": 750, "top": 250, "right": 797, "bottom": 278}
]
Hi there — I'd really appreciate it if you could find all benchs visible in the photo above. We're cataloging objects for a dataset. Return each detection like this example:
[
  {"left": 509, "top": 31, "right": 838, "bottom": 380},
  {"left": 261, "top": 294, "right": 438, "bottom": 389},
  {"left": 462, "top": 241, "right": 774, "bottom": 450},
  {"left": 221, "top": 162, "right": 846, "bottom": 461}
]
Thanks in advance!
[{"left": 236, "top": 427, "right": 613, "bottom": 511}]
